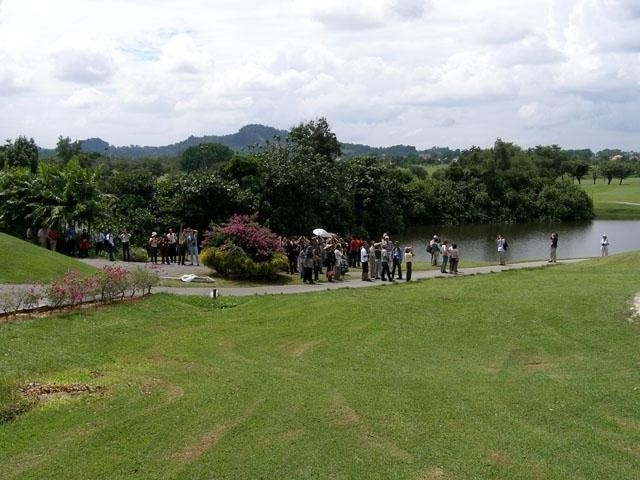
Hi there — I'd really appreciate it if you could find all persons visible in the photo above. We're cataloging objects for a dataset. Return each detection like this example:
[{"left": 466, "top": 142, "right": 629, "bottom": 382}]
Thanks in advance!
[
  {"left": 599, "top": 233, "right": 610, "bottom": 259},
  {"left": 493, "top": 231, "right": 509, "bottom": 267},
  {"left": 274, "top": 227, "right": 460, "bottom": 285},
  {"left": 547, "top": 230, "right": 560, "bottom": 265},
  {"left": 24, "top": 221, "right": 203, "bottom": 267}
]
